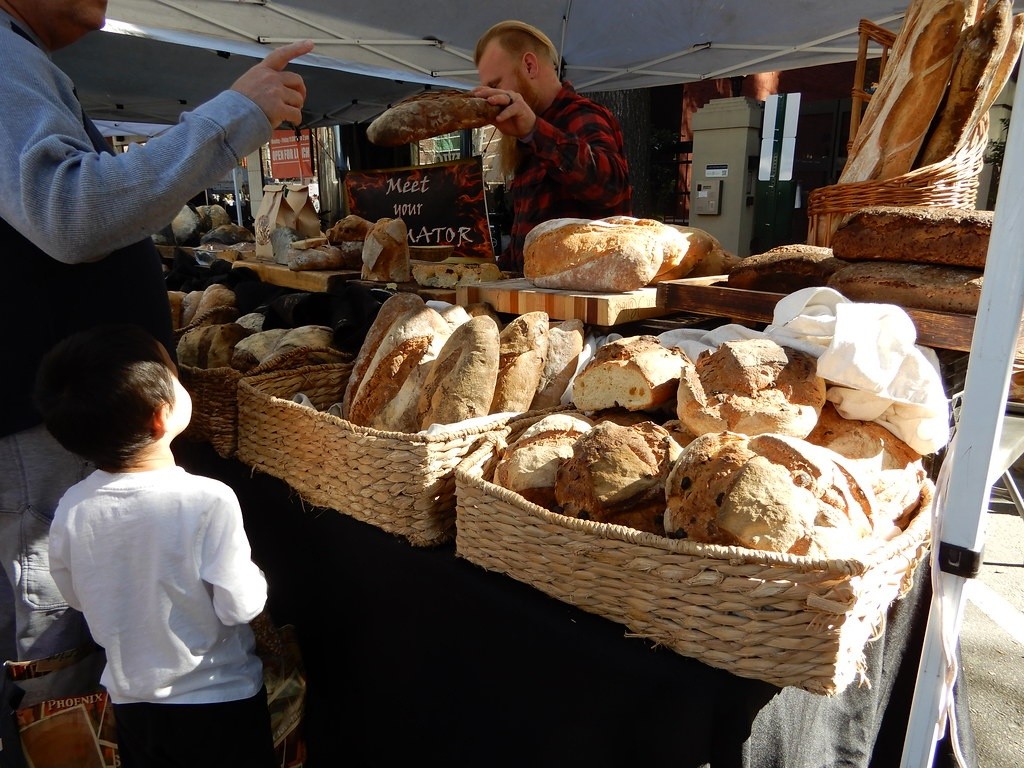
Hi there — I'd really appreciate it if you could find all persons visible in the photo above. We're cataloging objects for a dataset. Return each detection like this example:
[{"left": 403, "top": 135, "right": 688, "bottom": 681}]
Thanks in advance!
[
  {"left": 468, "top": 19, "right": 636, "bottom": 275},
  {"left": 0, "top": 0, "right": 317, "bottom": 711},
  {"left": 45, "top": 323, "right": 272, "bottom": 768}
]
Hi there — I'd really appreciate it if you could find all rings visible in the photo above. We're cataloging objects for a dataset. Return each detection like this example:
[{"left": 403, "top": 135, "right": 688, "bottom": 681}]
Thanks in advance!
[{"left": 506, "top": 93, "right": 513, "bottom": 105}]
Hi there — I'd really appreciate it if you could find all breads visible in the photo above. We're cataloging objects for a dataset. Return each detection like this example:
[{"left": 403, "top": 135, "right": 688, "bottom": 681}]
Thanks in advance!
[
  {"left": 366, "top": 89, "right": 505, "bottom": 145},
  {"left": 836, "top": 0, "right": 1024, "bottom": 184},
  {"left": 165, "top": 204, "right": 1024, "bottom": 558}
]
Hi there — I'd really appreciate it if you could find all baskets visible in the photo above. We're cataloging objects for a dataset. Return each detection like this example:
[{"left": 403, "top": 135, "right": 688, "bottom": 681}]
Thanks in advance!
[
  {"left": 173, "top": 308, "right": 356, "bottom": 456},
  {"left": 807, "top": 108, "right": 990, "bottom": 249},
  {"left": 454, "top": 397, "right": 939, "bottom": 694},
  {"left": 236, "top": 361, "right": 519, "bottom": 547}
]
